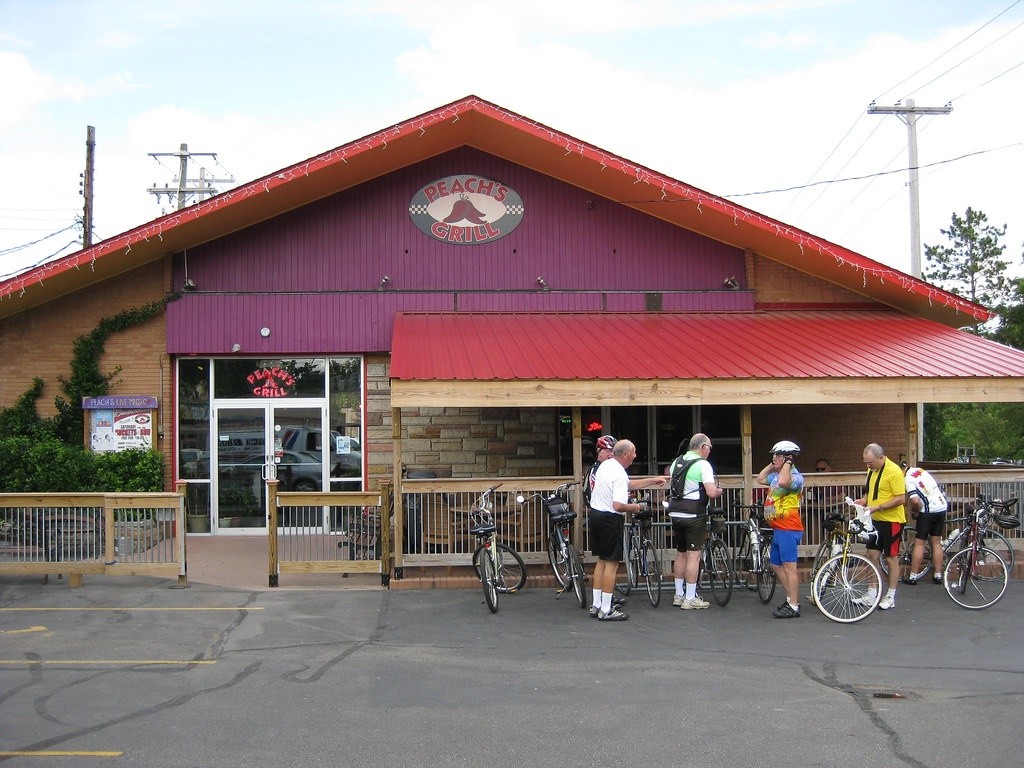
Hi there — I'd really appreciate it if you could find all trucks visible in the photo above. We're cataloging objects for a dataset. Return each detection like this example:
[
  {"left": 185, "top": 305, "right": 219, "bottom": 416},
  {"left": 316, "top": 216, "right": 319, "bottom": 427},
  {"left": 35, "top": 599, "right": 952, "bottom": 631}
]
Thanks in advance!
[{"left": 208, "top": 424, "right": 361, "bottom": 470}]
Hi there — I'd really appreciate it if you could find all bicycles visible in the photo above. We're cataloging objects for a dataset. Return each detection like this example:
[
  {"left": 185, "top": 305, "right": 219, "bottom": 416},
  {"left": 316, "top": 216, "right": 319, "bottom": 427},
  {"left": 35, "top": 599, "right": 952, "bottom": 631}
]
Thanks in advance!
[
  {"left": 520, "top": 481, "right": 589, "bottom": 608},
  {"left": 629, "top": 501, "right": 663, "bottom": 606},
  {"left": 880, "top": 493, "right": 1015, "bottom": 609},
  {"left": 697, "top": 505, "right": 734, "bottom": 607},
  {"left": 470, "top": 483, "right": 527, "bottom": 613},
  {"left": 809, "top": 510, "right": 884, "bottom": 624},
  {"left": 733, "top": 499, "right": 777, "bottom": 604}
]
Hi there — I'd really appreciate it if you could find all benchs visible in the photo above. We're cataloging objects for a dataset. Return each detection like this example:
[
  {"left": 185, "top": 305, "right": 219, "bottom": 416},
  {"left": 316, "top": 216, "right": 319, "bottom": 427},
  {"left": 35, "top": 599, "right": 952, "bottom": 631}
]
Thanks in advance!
[{"left": 3, "top": 510, "right": 118, "bottom": 585}]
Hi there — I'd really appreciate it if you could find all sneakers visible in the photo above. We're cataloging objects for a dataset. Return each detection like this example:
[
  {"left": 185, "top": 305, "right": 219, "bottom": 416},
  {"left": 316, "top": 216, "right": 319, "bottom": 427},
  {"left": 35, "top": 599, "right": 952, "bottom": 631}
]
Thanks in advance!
[
  {"left": 598, "top": 608, "right": 629, "bottom": 620},
  {"left": 775, "top": 598, "right": 800, "bottom": 618},
  {"left": 681, "top": 598, "right": 710, "bottom": 610},
  {"left": 673, "top": 594, "right": 686, "bottom": 606},
  {"left": 879, "top": 593, "right": 895, "bottom": 610},
  {"left": 611, "top": 603, "right": 619, "bottom": 610},
  {"left": 851, "top": 592, "right": 876, "bottom": 606},
  {"left": 901, "top": 574, "right": 917, "bottom": 585},
  {"left": 611, "top": 594, "right": 628, "bottom": 605},
  {"left": 932, "top": 573, "right": 942, "bottom": 586},
  {"left": 589, "top": 606, "right": 600, "bottom": 617}
]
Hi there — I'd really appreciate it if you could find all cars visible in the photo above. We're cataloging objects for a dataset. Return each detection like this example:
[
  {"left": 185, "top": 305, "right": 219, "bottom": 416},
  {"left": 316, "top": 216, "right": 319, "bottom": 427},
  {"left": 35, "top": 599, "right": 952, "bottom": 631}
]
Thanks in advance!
[
  {"left": 180, "top": 448, "right": 203, "bottom": 472},
  {"left": 221, "top": 450, "right": 322, "bottom": 492}
]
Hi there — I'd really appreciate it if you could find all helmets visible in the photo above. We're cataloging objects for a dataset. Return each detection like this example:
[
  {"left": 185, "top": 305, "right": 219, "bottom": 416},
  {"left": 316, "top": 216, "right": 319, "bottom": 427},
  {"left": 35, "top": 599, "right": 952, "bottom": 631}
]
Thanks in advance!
[
  {"left": 769, "top": 441, "right": 803, "bottom": 461},
  {"left": 995, "top": 512, "right": 1020, "bottom": 529},
  {"left": 597, "top": 435, "right": 617, "bottom": 449}
]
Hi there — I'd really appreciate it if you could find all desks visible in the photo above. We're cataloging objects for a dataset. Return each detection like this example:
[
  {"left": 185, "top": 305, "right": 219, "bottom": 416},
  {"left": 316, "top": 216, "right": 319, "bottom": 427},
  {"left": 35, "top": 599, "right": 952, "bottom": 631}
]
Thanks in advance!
[
  {"left": 948, "top": 497, "right": 978, "bottom": 507},
  {"left": 803, "top": 499, "right": 846, "bottom": 542},
  {"left": 448, "top": 504, "right": 520, "bottom": 551}
]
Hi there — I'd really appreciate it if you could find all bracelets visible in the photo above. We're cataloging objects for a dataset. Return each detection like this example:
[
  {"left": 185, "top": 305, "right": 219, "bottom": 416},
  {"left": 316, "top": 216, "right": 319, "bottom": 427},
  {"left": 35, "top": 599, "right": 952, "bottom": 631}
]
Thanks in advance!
[
  {"left": 785, "top": 461, "right": 792, "bottom": 465},
  {"left": 880, "top": 505, "right": 883, "bottom": 511}
]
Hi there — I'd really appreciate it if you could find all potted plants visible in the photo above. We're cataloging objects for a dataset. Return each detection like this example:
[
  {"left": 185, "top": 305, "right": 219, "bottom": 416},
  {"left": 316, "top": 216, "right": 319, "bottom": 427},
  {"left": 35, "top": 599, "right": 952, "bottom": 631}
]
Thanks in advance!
[{"left": 179, "top": 458, "right": 267, "bottom": 532}]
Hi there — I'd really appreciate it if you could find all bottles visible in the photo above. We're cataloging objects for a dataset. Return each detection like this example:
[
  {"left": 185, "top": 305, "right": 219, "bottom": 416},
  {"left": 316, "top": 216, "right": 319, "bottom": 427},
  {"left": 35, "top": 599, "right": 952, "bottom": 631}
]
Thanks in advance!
[
  {"left": 562, "top": 530, "right": 569, "bottom": 540},
  {"left": 485, "top": 542, "right": 492, "bottom": 559},
  {"left": 830, "top": 544, "right": 842, "bottom": 569},
  {"left": 943, "top": 528, "right": 959, "bottom": 546},
  {"left": 750, "top": 530, "right": 758, "bottom": 553}
]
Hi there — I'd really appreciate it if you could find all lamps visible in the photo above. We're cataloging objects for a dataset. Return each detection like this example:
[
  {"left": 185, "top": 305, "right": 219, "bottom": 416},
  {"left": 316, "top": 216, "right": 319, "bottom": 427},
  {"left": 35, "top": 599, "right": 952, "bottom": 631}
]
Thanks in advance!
[
  {"left": 181, "top": 279, "right": 197, "bottom": 291},
  {"left": 537, "top": 276, "right": 549, "bottom": 290},
  {"left": 724, "top": 277, "right": 740, "bottom": 290},
  {"left": 380, "top": 275, "right": 390, "bottom": 290}
]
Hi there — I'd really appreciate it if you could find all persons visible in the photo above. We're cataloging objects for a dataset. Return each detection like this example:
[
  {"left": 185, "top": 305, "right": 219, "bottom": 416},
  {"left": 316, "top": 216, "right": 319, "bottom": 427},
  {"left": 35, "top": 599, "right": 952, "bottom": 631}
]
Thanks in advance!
[
  {"left": 92, "top": 434, "right": 115, "bottom": 449},
  {"left": 587, "top": 439, "right": 673, "bottom": 620},
  {"left": 755, "top": 441, "right": 806, "bottom": 618},
  {"left": 811, "top": 459, "right": 849, "bottom": 522},
  {"left": 589, "top": 434, "right": 636, "bottom": 612},
  {"left": 850, "top": 442, "right": 907, "bottom": 609},
  {"left": 896, "top": 460, "right": 946, "bottom": 585},
  {"left": 668, "top": 433, "right": 723, "bottom": 609}
]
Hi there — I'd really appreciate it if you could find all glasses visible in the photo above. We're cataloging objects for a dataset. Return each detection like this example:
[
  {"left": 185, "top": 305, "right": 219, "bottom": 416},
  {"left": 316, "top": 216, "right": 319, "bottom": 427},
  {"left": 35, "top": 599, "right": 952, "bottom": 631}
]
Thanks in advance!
[{"left": 772, "top": 453, "right": 785, "bottom": 459}]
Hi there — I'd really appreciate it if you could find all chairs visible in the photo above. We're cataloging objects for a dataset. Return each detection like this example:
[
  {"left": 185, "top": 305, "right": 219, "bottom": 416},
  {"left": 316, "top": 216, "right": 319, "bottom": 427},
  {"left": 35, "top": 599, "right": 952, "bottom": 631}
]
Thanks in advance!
[
  {"left": 338, "top": 491, "right": 393, "bottom": 577},
  {"left": 945, "top": 485, "right": 980, "bottom": 532},
  {"left": 422, "top": 491, "right": 549, "bottom": 549}
]
